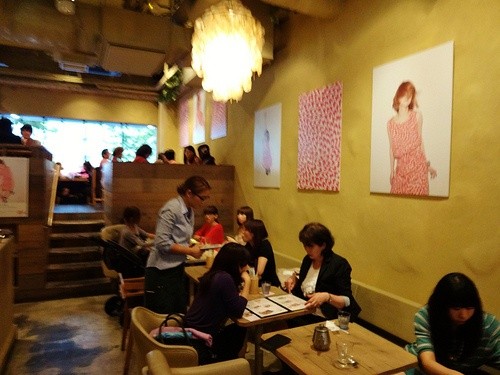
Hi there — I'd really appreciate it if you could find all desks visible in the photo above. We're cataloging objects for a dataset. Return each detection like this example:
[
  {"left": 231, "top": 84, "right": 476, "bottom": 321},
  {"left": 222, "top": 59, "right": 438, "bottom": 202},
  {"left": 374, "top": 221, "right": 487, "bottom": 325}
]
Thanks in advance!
[
  {"left": 184, "top": 264, "right": 208, "bottom": 284},
  {"left": 227, "top": 285, "right": 317, "bottom": 375},
  {"left": 260, "top": 316, "right": 417, "bottom": 375}
]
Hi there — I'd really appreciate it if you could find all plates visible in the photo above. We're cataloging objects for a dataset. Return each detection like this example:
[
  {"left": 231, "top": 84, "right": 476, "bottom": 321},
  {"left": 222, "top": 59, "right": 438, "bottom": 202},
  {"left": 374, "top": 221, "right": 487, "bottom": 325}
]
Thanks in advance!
[
  {"left": 267, "top": 294, "right": 311, "bottom": 311},
  {"left": 246, "top": 297, "right": 288, "bottom": 318}
]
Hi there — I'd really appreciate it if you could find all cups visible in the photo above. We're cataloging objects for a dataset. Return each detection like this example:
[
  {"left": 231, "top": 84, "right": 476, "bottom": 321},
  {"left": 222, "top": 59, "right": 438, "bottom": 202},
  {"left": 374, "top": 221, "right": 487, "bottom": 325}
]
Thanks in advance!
[
  {"left": 338, "top": 311, "right": 351, "bottom": 331},
  {"left": 336, "top": 339, "right": 353, "bottom": 368},
  {"left": 249, "top": 276, "right": 259, "bottom": 295},
  {"left": 262, "top": 281, "right": 271, "bottom": 296},
  {"left": 312, "top": 324, "right": 331, "bottom": 351}
]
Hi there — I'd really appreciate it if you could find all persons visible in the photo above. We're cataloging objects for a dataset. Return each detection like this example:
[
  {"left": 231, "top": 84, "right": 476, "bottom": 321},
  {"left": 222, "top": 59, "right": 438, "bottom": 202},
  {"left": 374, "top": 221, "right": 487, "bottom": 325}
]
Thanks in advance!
[
  {"left": 387, "top": 82, "right": 437, "bottom": 196},
  {"left": 84, "top": 162, "right": 94, "bottom": 180},
  {"left": 193, "top": 205, "right": 224, "bottom": 251},
  {"left": 112, "top": 147, "right": 124, "bottom": 162},
  {"left": 120, "top": 206, "right": 155, "bottom": 255},
  {"left": 100, "top": 149, "right": 110, "bottom": 166},
  {"left": 404, "top": 272, "right": 500, "bottom": 375},
  {"left": 164, "top": 150, "right": 176, "bottom": 164},
  {"left": 198, "top": 145, "right": 214, "bottom": 165},
  {"left": 185, "top": 146, "right": 200, "bottom": 165},
  {"left": 185, "top": 242, "right": 252, "bottom": 361},
  {"left": 0, "top": 118, "right": 21, "bottom": 144},
  {"left": 261, "top": 111, "right": 272, "bottom": 175},
  {"left": 134, "top": 145, "right": 152, "bottom": 163},
  {"left": 21, "top": 124, "right": 41, "bottom": 145},
  {"left": 285, "top": 222, "right": 361, "bottom": 327},
  {"left": 57, "top": 162, "right": 63, "bottom": 197},
  {"left": 242, "top": 219, "right": 281, "bottom": 287},
  {"left": 145, "top": 175, "right": 211, "bottom": 314},
  {"left": 227, "top": 206, "right": 254, "bottom": 245}
]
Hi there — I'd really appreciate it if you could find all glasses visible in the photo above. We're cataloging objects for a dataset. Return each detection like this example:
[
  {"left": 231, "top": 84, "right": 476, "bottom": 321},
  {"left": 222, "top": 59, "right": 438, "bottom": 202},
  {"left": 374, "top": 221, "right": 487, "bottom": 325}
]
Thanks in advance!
[{"left": 195, "top": 192, "right": 210, "bottom": 201}]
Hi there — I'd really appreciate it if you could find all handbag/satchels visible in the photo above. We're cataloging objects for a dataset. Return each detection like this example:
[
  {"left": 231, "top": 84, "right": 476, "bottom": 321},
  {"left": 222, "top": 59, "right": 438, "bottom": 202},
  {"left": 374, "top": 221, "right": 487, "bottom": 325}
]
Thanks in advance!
[{"left": 152, "top": 312, "right": 206, "bottom": 364}]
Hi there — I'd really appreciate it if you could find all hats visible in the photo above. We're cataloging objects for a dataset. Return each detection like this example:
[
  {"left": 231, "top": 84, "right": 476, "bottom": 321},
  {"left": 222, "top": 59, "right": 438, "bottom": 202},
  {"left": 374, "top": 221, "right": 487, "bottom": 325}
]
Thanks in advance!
[{"left": 21, "top": 123, "right": 32, "bottom": 133}]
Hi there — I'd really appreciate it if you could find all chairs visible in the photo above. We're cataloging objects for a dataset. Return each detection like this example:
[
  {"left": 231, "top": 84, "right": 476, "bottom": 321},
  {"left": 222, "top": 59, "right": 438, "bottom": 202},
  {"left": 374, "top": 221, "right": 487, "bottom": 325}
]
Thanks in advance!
[
  {"left": 100, "top": 260, "right": 251, "bottom": 375},
  {"left": 89, "top": 167, "right": 103, "bottom": 208}
]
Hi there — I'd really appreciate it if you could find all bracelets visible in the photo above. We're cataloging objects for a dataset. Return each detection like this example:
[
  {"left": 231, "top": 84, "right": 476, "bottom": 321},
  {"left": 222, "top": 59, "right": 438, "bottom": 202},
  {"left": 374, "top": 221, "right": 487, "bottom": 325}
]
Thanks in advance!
[{"left": 327, "top": 293, "right": 332, "bottom": 304}]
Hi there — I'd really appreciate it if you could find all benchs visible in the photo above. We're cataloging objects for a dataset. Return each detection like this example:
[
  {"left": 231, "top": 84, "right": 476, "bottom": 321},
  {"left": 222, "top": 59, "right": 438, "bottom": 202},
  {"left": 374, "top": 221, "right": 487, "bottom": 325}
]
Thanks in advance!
[{"left": 272, "top": 250, "right": 419, "bottom": 349}]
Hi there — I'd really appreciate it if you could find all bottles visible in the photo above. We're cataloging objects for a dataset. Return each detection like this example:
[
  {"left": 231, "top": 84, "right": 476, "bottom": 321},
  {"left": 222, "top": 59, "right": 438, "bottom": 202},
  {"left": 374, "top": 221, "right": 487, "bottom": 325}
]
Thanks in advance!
[{"left": 206, "top": 249, "right": 215, "bottom": 269}]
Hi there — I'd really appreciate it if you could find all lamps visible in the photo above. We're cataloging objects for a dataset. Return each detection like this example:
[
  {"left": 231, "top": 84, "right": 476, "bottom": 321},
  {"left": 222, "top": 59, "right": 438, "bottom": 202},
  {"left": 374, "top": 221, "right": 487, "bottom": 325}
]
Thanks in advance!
[
  {"left": 54, "top": 0, "right": 75, "bottom": 16},
  {"left": 191, "top": 0, "right": 265, "bottom": 104}
]
